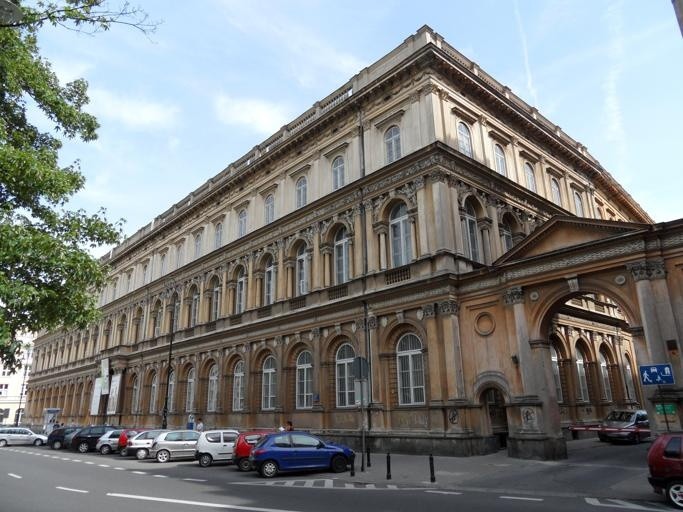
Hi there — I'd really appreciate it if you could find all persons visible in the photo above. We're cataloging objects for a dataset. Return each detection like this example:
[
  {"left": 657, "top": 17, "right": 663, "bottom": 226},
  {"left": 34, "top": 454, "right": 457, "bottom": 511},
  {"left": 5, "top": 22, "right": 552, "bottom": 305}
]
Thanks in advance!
[
  {"left": 42, "top": 418, "right": 75, "bottom": 436},
  {"left": 285, "top": 421, "right": 294, "bottom": 432},
  {"left": 610, "top": 412, "right": 632, "bottom": 422},
  {"left": 196, "top": 417, "right": 204, "bottom": 433}
]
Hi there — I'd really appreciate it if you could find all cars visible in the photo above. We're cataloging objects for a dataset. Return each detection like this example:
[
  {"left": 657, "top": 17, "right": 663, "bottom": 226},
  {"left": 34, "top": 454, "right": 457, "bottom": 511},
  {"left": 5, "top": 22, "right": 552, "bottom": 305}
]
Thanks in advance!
[
  {"left": 598, "top": 408, "right": 651, "bottom": 446},
  {"left": 48, "top": 425, "right": 201, "bottom": 462},
  {"left": 196, "top": 429, "right": 355, "bottom": 478},
  {"left": 645, "top": 434, "right": 683, "bottom": 509}
]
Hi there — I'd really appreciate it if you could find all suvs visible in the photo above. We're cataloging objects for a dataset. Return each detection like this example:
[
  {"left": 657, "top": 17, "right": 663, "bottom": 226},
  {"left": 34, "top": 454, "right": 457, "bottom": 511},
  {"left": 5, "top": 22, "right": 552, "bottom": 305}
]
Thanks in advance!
[{"left": 0, "top": 427, "right": 49, "bottom": 446}]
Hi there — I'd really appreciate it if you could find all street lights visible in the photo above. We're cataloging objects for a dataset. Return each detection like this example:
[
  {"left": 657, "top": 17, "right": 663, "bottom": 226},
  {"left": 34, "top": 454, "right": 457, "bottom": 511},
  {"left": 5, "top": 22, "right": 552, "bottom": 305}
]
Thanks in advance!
[{"left": 161, "top": 277, "right": 176, "bottom": 429}]
